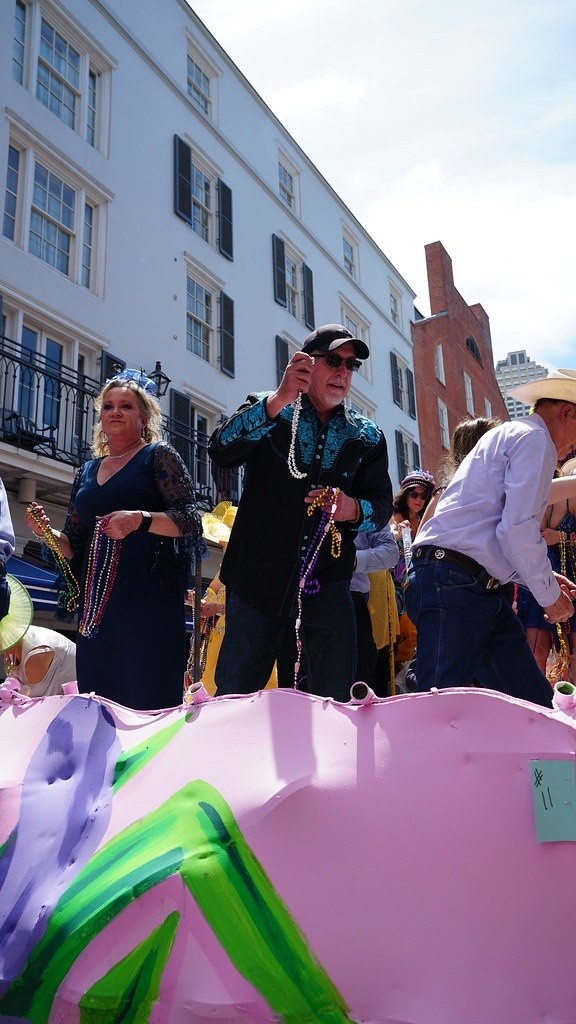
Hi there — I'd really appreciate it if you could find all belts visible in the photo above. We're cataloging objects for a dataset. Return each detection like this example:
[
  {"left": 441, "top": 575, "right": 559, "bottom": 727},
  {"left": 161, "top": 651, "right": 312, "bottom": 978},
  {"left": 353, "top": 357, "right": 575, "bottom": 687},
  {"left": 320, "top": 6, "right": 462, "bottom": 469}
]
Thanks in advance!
[{"left": 411, "top": 545, "right": 503, "bottom": 589}]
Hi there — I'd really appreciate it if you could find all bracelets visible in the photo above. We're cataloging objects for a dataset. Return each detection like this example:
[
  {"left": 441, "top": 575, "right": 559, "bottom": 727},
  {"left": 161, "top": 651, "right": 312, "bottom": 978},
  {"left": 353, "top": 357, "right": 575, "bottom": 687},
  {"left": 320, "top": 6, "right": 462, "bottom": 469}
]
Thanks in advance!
[
  {"left": 136, "top": 510, "right": 152, "bottom": 533},
  {"left": 220, "top": 605, "right": 224, "bottom": 614}
]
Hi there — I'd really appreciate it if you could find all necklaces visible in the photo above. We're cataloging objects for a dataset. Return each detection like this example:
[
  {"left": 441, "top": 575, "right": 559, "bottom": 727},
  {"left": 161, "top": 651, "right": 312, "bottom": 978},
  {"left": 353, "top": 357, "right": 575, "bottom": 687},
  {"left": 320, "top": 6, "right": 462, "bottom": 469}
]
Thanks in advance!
[
  {"left": 26, "top": 505, "right": 80, "bottom": 613},
  {"left": 545, "top": 530, "right": 576, "bottom": 680},
  {"left": 77, "top": 517, "right": 121, "bottom": 640},
  {"left": 288, "top": 356, "right": 315, "bottom": 480},
  {"left": 187, "top": 593, "right": 215, "bottom": 682},
  {"left": 294, "top": 485, "right": 341, "bottom": 688},
  {"left": 108, "top": 448, "right": 134, "bottom": 459}
]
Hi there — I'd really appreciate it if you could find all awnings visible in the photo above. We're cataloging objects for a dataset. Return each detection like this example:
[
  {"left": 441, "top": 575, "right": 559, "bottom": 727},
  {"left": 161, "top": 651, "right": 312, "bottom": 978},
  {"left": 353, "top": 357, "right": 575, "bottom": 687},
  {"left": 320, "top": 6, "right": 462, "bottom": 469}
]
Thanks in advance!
[{"left": 1, "top": 556, "right": 79, "bottom": 614}]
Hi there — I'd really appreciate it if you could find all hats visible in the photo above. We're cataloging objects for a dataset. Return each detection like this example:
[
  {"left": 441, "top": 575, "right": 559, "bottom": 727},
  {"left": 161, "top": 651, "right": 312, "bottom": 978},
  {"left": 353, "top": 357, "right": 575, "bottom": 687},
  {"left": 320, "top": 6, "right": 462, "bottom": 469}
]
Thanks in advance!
[
  {"left": 401, "top": 469, "right": 436, "bottom": 494},
  {"left": 202, "top": 500, "right": 238, "bottom": 544},
  {"left": 110, "top": 368, "right": 158, "bottom": 401},
  {"left": 300, "top": 324, "right": 370, "bottom": 359},
  {"left": 508, "top": 368, "right": 576, "bottom": 407},
  {"left": 0, "top": 573, "right": 33, "bottom": 650}
]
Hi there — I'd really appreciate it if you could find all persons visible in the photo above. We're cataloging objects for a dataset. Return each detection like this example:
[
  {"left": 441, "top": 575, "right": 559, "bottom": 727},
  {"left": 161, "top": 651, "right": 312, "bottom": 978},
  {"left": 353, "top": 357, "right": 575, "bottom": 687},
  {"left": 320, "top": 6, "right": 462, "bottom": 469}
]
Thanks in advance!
[
  {"left": 400, "top": 368, "right": 576, "bottom": 708},
  {"left": 206, "top": 324, "right": 395, "bottom": 702},
  {"left": 416, "top": 417, "right": 504, "bottom": 535},
  {"left": 184, "top": 500, "right": 277, "bottom": 696},
  {"left": 0, "top": 478, "right": 17, "bottom": 619},
  {"left": 0, "top": 572, "right": 77, "bottom": 697},
  {"left": 517, "top": 456, "right": 576, "bottom": 686},
  {"left": 25, "top": 366, "right": 202, "bottom": 710},
  {"left": 349, "top": 470, "right": 434, "bottom": 699}
]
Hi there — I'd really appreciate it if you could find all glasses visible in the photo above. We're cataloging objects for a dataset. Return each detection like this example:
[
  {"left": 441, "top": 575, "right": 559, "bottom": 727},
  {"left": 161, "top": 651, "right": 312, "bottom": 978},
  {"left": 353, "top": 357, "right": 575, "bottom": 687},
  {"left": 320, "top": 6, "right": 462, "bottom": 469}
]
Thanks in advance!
[
  {"left": 410, "top": 491, "right": 428, "bottom": 500},
  {"left": 309, "top": 352, "right": 362, "bottom": 371}
]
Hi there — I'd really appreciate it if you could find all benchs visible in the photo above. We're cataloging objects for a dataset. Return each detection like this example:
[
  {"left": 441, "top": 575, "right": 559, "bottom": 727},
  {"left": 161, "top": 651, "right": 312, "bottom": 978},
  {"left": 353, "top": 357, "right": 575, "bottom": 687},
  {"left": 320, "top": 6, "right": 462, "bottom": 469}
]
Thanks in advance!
[{"left": 0, "top": 408, "right": 57, "bottom": 458}]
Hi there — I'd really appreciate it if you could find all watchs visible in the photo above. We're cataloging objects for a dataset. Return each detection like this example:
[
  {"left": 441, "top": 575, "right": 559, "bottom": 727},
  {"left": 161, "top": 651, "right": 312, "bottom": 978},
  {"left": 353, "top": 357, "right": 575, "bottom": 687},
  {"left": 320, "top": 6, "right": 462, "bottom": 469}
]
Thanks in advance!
[{"left": 565, "top": 531, "right": 571, "bottom": 545}]
